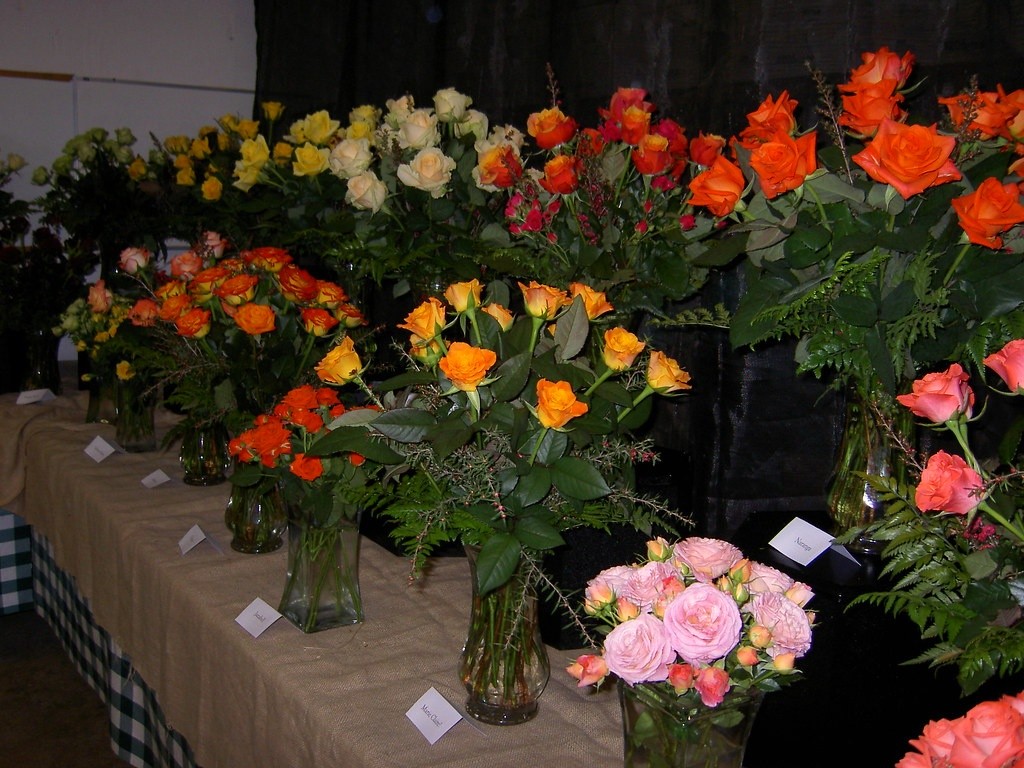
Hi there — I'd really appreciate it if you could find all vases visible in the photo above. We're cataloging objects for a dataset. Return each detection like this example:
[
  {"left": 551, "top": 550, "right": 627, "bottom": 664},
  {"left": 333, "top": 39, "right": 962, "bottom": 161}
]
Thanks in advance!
[
  {"left": 617, "top": 677, "right": 765, "bottom": 767},
  {"left": 28, "top": 334, "right": 62, "bottom": 394},
  {"left": 224, "top": 455, "right": 287, "bottom": 554},
  {"left": 179, "top": 411, "right": 232, "bottom": 486},
  {"left": 278, "top": 502, "right": 364, "bottom": 634},
  {"left": 114, "top": 373, "right": 158, "bottom": 453},
  {"left": 457, "top": 540, "right": 550, "bottom": 725},
  {"left": 828, "top": 379, "right": 916, "bottom": 554},
  {"left": 87, "top": 360, "right": 118, "bottom": 424}
]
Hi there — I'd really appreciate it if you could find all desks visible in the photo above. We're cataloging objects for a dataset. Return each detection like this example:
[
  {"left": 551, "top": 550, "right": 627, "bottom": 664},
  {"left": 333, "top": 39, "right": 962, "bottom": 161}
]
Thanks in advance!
[{"left": 2, "top": 392, "right": 625, "bottom": 767}]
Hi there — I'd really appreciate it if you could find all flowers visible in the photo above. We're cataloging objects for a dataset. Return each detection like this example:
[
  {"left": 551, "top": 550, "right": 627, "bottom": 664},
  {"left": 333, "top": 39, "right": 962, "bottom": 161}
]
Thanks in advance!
[{"left": 1, "top": 46, "right": 1024, "bottom": 768}]
